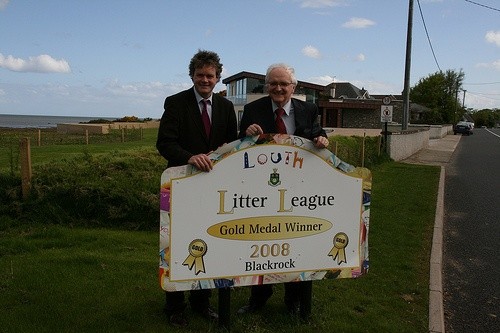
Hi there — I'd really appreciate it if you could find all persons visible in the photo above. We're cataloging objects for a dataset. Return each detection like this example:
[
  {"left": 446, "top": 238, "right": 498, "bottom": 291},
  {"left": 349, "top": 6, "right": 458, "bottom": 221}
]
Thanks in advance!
[
  {"left": 239, "top": 63, "right": 330, "bottom": 325},
  {"left": 155, "top": 49, "right": 239, "bottom": 328}
]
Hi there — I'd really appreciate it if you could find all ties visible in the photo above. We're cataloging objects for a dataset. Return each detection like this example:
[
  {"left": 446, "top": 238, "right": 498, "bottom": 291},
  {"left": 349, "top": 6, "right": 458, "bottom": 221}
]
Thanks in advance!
[
  {"left": 200, "top": 99, "right": 211, "bottom": 138},
  {"left": 273, "top": 107, "right": 287, "bottom": 134}
]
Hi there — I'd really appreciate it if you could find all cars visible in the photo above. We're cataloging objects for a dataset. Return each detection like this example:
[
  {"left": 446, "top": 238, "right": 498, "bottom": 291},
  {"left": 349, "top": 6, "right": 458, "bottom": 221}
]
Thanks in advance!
[{"left": 454, "top": 121, "right": 474, "bottom": 136}]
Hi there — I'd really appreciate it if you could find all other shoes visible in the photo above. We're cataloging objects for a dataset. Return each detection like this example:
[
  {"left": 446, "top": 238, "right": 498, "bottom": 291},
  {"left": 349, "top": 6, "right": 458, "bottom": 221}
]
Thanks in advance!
[
  {"left": 167, "top": 306, "right": 188, "bottom": 327},
  {"left": 284, "top": 296, "right": 300, "bottom": 318},
  {"left": 191, "top": 304, "right": 219, "bottom": 320},
  {"left": 235, "top": 299, "right": 266, "bottom": 314}
]
